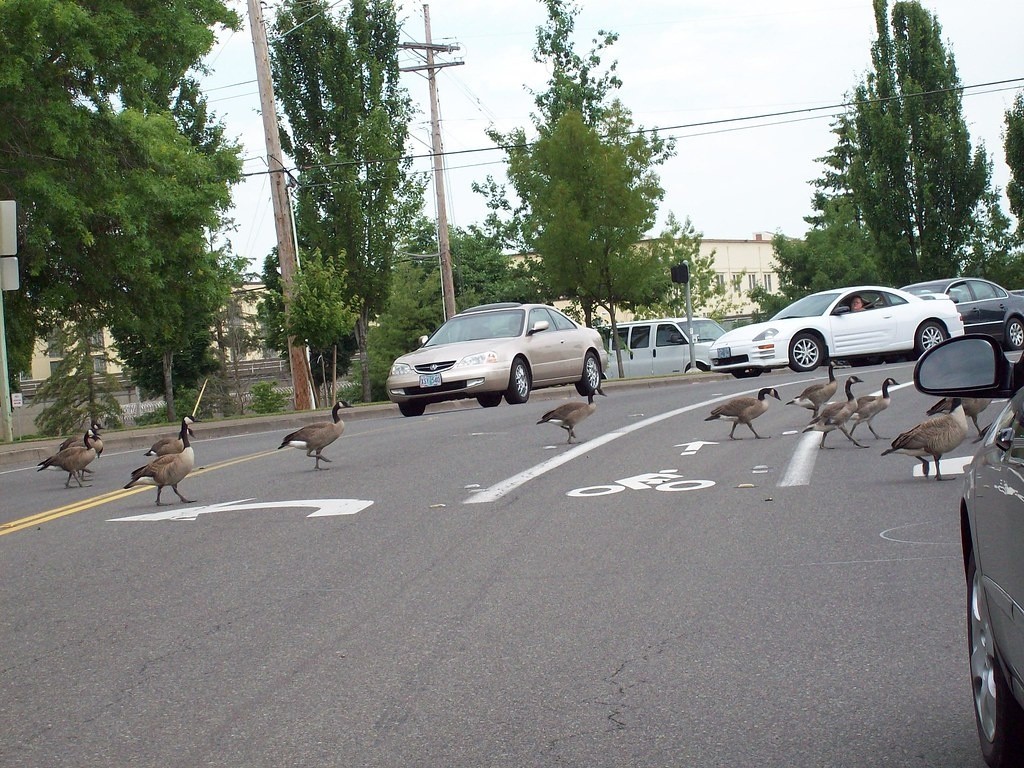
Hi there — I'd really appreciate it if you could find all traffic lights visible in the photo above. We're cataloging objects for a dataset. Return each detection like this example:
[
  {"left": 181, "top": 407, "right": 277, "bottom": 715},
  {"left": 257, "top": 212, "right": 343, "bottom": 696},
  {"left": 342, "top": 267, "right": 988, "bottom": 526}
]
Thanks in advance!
[{"left": 671, "top": 264, "right": 688, "bottom": 284}]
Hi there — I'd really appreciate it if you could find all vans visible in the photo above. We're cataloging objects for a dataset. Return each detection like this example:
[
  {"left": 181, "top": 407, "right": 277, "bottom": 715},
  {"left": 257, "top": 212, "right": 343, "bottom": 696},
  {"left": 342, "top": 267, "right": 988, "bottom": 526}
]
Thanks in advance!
[{"left": 593, "top": 319, "right": 728, "bottom": 381}]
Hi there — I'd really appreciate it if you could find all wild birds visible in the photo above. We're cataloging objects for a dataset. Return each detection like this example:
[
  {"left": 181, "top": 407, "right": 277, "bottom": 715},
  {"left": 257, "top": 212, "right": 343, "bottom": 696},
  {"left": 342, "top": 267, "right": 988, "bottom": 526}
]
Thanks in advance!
[
  {"left": 537, "top": 388, "right": 608, "bottom": 444},
  {"left": 37, "top": 425, "right": 101, "bottom": 489},
  {"left": 803, "top": 375, "right": 871, "bottom": 449},
  {"left": 879, "top": 399, "right": 970, "bottom": 482},
  {"left": 277, "top": 400, "right": 355, "bottom": 472},
  {"left": 704, "top": 387, "right": 782, "bottom": 439},
  {"left": 784, "top": 359, "right": 843, "bottom": 418},
  {"left": 849, "top": 377, "right": 901, "bottom": 440},
  {"left": 143, "top": 428, "right": 196, "bottom": 457},
  {"left": 122, "top": 416, "right": 203, "bottom": 506},
  {"left": 927, "top": 396, "right": 993, "bottom": 436},
  {"left": 56, "top": 421, "right": 105, "bottom": 459}
]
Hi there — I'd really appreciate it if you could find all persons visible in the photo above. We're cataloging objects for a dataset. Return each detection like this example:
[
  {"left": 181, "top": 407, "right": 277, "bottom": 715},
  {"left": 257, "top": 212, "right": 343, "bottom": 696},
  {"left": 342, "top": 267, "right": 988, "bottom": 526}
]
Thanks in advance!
[
  {"left": 850, "top": 295, "right": 866, "bottom": 312},
  {"left": 508, "top": 315, "right": 522, "bottom": 336}
]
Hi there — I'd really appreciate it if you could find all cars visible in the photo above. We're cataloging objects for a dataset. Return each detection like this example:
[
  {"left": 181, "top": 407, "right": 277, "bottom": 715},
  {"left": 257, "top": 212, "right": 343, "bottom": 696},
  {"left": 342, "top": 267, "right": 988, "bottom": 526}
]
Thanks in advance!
[
  {"left": 707, "top": 286, "right": 965, "bottom": 378},
  {"left": 913, "top": 333, "right": 1024, "bottom": 768},
  {"left": 872, "top": 277, "right": 1024, "bottom": 363},
  {"left": 385, "top": 302, "right": 608, "bottom": 417}
]
